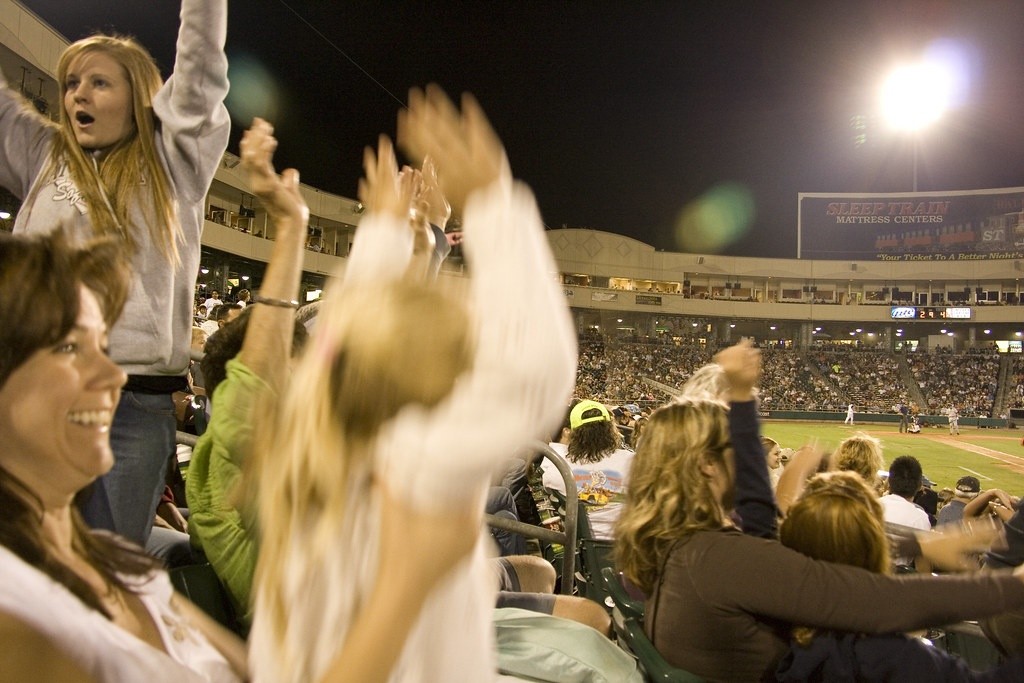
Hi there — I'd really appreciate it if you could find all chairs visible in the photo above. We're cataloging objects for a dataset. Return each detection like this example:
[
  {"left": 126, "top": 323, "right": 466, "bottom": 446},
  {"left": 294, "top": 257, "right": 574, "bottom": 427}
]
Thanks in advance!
[
  {"left": 190, "top": 393, "right": 206, "bottom": 436},
  {"left": 167, "top": 544, "right": 230, "bottom": 624},
  {"left": 898, "top": 562, "right": 1002, "bottom": 670},
  {"left": 548, "top": 486, "right": 706, "bottom": 683}
]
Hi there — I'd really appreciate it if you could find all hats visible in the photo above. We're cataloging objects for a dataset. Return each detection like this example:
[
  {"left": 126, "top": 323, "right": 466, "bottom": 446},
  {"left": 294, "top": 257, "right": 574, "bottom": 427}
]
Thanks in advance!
[
  {"left": 613, "top": 407, "right": 628, "bottom": 417},
  {"left": 922, "top": 475, "right": 937, "bottom": 487},
  {"left": 569, "top": 400, "right": 609, "bottom": 429},
  {"left": 956, "top": 476, "right": 981, "bottom": 492},
  {"left": 780, "top": 448, "right": 794, "bottom": 461}
]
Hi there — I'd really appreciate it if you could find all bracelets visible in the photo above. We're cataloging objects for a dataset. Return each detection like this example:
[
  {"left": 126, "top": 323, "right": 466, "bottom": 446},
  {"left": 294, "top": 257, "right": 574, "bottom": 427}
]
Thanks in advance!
[{"left": 255, "top": 295, "right": 301, "bottom": 310}]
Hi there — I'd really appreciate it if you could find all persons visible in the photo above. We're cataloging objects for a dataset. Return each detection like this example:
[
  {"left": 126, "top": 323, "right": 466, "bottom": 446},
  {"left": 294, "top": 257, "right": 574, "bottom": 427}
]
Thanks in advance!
[
  {"left": 0, "top": 0, "right": 233, "bottom": 546},
  {"left": 0, "top": 82, "right": 1024, "bottom": 683}
]
